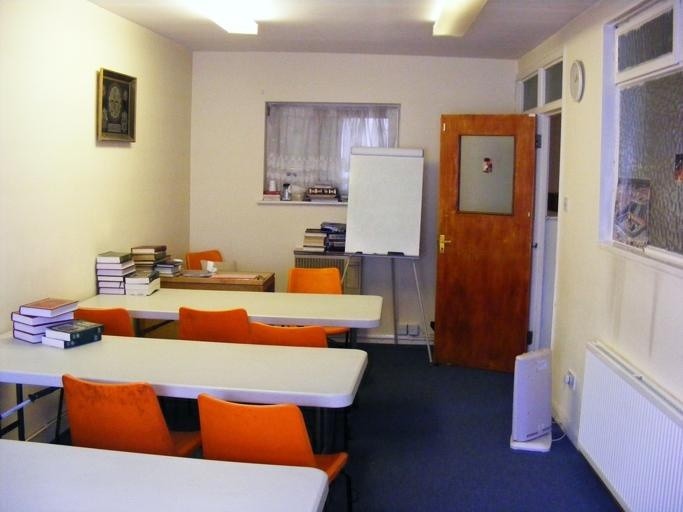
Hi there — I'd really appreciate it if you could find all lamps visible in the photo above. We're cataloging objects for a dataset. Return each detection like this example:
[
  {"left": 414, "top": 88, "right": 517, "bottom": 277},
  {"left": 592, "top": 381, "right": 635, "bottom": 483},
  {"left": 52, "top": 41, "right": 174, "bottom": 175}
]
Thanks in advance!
[{"left": 431, "top": 0, "right": 489, "bottom": 38}]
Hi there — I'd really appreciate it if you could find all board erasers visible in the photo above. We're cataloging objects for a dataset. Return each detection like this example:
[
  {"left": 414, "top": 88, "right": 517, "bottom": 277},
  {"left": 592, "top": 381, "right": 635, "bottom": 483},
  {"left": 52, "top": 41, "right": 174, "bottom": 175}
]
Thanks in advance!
[{"left": 388, "top": 252, "right": 403, "bottom": 256}]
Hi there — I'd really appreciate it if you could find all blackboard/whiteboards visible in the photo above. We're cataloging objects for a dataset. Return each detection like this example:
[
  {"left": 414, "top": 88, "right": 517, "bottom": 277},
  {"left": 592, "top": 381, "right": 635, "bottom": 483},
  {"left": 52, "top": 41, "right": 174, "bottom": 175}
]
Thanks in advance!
[{"left": 344, "top": 147, "right": 424, "bottom": 259}]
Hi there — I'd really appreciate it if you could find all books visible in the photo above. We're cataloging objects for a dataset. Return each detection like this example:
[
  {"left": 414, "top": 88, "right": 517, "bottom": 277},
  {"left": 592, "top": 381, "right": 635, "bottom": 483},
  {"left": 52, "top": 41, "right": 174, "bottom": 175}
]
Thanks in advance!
[
  {"left": 9, "top": 296, "right": 105, "bottom": 349},
  {"left": 301, "top": 221, "right": 346, "bottom": 254},
  {"left": 95, "top": 244, "right": 213, "bottom": 298}
]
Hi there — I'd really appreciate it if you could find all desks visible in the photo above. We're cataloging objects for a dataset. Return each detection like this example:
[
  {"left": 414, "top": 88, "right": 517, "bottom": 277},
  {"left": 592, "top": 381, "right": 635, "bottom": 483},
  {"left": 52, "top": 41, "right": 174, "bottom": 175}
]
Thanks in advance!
[
  {"left": 1, "top": 438, "right": 329, "bottom": 512},
  {"left": 294, "top": 251, "right": 362, "bottom": 293},
  {"left": 159, "top": 268, "right": 278, "bottom": 292},
  {"left": 1, "top": 335, "right": 369, "bottom": 452},
  {"left": 76, "top": 286, "right": 384, "bottom": 348}
]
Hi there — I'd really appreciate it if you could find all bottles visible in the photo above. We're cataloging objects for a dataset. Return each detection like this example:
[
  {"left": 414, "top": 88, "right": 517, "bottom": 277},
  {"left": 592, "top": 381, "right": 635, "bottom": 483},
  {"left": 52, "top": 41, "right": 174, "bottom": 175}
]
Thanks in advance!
[
  {"left": 281, "top": 183, "right": 292, "bottom": 201},
  {"left": 269, "top": 180, "right": 277, "bottom": 192}
]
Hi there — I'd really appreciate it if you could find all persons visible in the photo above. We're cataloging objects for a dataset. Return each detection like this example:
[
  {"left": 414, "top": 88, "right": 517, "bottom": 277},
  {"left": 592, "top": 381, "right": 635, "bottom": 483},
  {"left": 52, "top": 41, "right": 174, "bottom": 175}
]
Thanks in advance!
[{"left": 481, "top": 156, "right": 492, "bottom": 173}]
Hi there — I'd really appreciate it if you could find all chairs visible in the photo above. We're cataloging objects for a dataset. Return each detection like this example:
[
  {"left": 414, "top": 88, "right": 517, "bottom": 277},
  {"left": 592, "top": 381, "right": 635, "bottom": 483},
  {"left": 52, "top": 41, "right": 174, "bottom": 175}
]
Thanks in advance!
[
  {"left": 251, "top": 321, "right": 329, "bottom": 453},
  {"left": 196, "top": 394, "right": 351, "bottom": 512},
  {"left": 286, "top": 267, "right": 352, "bottom": 346},
  {"left": 74, "top": 306, "right": 136, "bottom": 337},
  {"left": 61, "top": 373, "right": 200, "bottom": 457},
  {"left": 183, "top": 251, "right": 223, "bottom": 270},
  {"left": 178, "top": 307, "right": 251, "bottom": 342}
]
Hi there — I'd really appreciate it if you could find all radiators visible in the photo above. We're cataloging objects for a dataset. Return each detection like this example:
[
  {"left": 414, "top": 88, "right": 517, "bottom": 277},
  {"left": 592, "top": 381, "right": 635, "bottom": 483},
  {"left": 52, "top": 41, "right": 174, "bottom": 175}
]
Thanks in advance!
[{"left": 574, "top": 338, "right": 683, "bottom": 512}]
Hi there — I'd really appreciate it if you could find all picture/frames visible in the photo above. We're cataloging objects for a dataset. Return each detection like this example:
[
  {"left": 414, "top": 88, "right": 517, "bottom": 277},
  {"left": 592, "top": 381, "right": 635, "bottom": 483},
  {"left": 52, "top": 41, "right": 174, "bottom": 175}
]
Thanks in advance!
[{"left": 97, "top": 67, "right": 137, "bottom": 143}]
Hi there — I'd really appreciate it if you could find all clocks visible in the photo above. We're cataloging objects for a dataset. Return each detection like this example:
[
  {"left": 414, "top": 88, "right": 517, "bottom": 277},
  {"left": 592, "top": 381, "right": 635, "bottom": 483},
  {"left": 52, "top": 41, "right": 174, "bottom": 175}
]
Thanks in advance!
[{"left": 570, "top": 60, "right": 584, "bottom": 102}]
